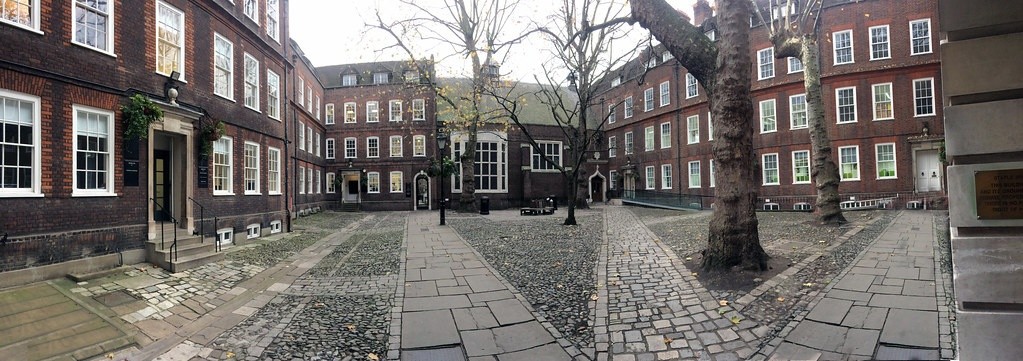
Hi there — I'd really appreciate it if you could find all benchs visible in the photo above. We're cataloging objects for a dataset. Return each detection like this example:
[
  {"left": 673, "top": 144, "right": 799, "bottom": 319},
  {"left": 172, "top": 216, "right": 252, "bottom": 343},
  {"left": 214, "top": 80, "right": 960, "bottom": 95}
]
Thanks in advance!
[{"left": 520, "top": 207, "right": 554, "bottom": 215}]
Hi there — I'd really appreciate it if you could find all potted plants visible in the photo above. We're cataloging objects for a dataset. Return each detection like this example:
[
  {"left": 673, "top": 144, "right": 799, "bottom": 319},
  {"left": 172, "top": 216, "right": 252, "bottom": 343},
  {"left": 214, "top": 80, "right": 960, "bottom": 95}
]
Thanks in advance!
[
  {"left": 199, "top": 116, "right": 225, "bottom": 156},
  {"left": 120, "top": 95, "right": 161, "bottom": 140}
]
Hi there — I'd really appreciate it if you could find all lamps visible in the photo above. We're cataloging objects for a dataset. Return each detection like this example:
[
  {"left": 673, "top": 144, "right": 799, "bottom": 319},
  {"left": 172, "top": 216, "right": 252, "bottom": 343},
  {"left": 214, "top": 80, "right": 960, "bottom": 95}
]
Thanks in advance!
[
  {"left": 923, "top": 127, "right": 928, "bottom": 132},
  {"left": 171, "top": 71, "right": 180, "bottom": 82},
  {"left": 350, "top": 161, "right": 352, "bottom": 164}
]
